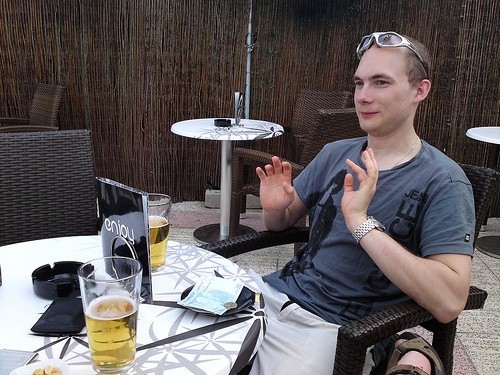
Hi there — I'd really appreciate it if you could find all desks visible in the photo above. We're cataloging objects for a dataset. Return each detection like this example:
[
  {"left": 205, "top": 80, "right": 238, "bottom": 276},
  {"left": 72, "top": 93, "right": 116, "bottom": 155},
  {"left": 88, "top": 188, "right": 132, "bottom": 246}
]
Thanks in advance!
[
  {"left": 0, "top": 234, "right": 269, "bottom": 375},
  {"left": 466, "top": 126, "right": 500, "bottom": 259},
  {"left": 171, "top": 117, "right": 286, "bottom": 244}
]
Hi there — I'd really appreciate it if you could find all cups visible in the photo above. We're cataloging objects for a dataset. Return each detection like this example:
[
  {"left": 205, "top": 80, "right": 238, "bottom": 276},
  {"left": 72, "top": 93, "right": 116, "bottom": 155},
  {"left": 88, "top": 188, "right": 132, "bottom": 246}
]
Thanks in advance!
[
  {"left": 145, "top": 192, "right": 171, "bottom": 272},
  {"left": 78, "top": 255, "right": 140, "bottom": 374}
]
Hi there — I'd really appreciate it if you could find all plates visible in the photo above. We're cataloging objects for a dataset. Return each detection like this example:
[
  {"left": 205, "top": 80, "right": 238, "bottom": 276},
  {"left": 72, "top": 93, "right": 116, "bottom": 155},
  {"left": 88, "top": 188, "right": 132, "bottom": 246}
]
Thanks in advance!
[
  {"left": 8, "top": 357, "right": 70, "bottom": 375},
  {"left": 180, "top": 278, "right": 254, "bottom": 316}
]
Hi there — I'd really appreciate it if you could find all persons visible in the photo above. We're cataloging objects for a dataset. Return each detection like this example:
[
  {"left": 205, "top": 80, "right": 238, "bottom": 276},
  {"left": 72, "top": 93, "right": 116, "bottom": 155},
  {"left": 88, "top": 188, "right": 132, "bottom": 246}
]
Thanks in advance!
[
  {"left": 366, "top": 330, "right": 447, "bottom": 375},
  {"left": 248, "top": 32, "right": 476, "bottom": 375}
]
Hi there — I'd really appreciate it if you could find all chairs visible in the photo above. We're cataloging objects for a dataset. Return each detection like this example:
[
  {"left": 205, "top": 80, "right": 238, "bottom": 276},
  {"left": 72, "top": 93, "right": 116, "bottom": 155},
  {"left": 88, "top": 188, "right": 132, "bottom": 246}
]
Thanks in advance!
[
  {"left": 0, "top": 82, "right": 65, "bottom": 133},
  {"left": 229, "top": 107, "right": 365, "bottom": 256},
  {"left": 199, "top": 162, "right": 500, "bottom": 375},
  {"left": 0, "top": 129, "right": 99, "bottom": 246},
  {"left": 282, "top": 87, "right": 355, "bottom": 164}
]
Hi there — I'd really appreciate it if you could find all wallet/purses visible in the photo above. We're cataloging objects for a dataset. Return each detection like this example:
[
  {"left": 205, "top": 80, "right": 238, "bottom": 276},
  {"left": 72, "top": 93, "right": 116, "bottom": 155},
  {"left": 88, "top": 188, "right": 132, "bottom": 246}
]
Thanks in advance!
[{"left": 30, "top": 296, "right": 86, "bottom": 334}]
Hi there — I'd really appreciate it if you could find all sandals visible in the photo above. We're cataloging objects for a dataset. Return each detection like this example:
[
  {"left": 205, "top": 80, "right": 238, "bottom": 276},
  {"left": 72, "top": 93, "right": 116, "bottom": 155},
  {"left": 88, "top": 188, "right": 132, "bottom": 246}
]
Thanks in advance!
[{"left": 385, "top": 332, "right": 444, "bottom": 375}]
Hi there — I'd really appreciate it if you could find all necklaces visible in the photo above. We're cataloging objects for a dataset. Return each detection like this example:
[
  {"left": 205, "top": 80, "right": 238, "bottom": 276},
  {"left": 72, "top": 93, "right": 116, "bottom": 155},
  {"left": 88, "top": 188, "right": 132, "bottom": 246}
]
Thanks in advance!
[{"left": 389, "top": 137, "right": 419, "bottom": 167}]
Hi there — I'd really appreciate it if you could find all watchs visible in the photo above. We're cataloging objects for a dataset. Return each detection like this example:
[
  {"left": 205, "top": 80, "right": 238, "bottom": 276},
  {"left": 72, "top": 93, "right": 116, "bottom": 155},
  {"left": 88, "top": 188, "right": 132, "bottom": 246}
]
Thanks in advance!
[{"left": 352, "top": 216, "right": 386, "bottom": 244}]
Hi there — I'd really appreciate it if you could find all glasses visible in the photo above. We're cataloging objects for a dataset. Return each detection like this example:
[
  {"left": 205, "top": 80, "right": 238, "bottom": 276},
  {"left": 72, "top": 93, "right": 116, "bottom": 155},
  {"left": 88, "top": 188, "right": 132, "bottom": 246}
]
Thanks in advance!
[{"left": 356, "top": 31, "right": 429, "bottom": 78}]
